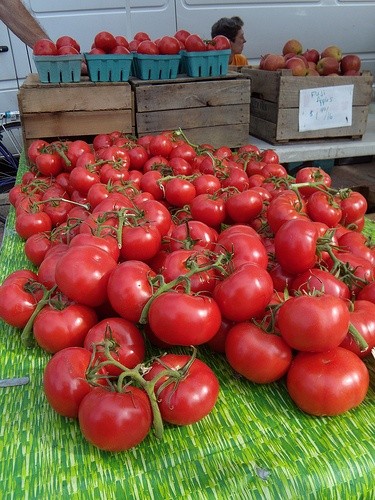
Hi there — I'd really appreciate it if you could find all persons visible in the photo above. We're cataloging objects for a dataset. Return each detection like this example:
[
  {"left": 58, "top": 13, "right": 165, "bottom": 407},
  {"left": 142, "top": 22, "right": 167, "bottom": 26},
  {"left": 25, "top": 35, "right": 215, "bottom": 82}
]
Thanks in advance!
[
  {"left": 0, "top": 0, "right": 88, "bottom": 74},
  {"left": 211, "top": 17, "right": 249, "bottom": 68}
]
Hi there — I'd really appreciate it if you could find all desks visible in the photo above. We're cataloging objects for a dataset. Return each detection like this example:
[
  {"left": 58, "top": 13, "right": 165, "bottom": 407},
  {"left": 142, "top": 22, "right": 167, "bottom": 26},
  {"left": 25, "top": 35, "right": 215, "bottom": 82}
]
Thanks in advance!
[{"left": 0, "top": 113, "right": 375, "bottom": 500}]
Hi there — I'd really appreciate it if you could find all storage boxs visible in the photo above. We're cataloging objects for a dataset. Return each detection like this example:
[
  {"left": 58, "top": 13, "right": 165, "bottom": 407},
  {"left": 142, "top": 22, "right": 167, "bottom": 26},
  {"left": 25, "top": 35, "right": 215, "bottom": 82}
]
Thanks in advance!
[{"left": 17, "top": 49, "right": 374, "bottom": 166}]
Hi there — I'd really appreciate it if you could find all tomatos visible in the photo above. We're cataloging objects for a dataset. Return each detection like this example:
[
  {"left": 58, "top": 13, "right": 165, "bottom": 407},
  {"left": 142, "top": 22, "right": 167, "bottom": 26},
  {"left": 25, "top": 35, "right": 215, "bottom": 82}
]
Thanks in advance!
[
  {"left": 34, "top": 30, "right": 231, "bottom": 56},
  {"left": 0, "top": 131, "right": 375, "bottom": 455}
]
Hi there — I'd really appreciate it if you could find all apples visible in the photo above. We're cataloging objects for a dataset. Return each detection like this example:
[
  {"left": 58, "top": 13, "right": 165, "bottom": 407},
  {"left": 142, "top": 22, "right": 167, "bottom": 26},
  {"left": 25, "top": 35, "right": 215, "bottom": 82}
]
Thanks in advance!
[{"left": 258, "top": 39, "right": 362, "bottom": 78}]
